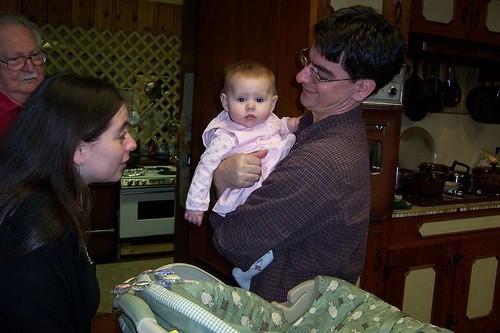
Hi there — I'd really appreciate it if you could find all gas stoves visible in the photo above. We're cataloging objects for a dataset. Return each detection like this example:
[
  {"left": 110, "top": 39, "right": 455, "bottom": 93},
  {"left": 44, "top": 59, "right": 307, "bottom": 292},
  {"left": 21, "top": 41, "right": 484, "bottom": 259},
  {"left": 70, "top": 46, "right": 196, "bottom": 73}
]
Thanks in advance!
[{"left": 121, "top": 164, "right": 177, "bottom": 187}]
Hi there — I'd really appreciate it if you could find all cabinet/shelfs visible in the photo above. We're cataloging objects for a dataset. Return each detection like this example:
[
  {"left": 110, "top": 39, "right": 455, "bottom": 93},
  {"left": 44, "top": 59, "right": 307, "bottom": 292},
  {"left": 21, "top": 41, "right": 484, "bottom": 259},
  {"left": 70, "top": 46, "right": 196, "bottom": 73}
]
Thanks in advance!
[
  {"left": 384, "top": 208, "right": 500, "bottom": 333},
  {"left": 309, "top": 0, "right": 411, "bottom": 67},
  {"left": 358, "top": 220, "right": 386, "bottom": 301},
  {"left": 409, "top": 0, "right": 500, "bottom": 60},
  {"left": 0, "top": 0, "right": 184, "bottom": 63}
]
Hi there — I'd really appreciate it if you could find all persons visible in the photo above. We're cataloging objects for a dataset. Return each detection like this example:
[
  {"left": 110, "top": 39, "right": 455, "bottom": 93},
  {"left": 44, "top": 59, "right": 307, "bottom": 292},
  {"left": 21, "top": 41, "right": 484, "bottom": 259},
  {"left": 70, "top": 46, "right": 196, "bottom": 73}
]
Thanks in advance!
[
  {"left": 184, "top": 61, "right": 305, "bottom": 292},
  {"left": 0, "top": 71, "right": 137, "bottom": 333},
  {"left": 207, "top": 4, "right": 405, "bottom": 304},
  {"left": 0, "top": 17, "right": 46, "bottom": 136}
]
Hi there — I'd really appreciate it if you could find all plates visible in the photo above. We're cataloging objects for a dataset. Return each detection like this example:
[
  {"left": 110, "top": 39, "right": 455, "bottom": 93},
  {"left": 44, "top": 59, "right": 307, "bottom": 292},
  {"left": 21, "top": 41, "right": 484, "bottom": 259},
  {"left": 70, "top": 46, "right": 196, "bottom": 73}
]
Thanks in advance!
[{"left": 397, "top": 125, "right": 438, "bottom": 173}]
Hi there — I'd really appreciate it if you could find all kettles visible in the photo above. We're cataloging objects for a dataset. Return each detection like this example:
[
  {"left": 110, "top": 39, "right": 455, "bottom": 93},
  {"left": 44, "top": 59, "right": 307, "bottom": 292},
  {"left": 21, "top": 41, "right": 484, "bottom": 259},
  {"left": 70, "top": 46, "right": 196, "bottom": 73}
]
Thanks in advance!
[{"left": 443, "top": 159, "right": 472, "bottom": 197}]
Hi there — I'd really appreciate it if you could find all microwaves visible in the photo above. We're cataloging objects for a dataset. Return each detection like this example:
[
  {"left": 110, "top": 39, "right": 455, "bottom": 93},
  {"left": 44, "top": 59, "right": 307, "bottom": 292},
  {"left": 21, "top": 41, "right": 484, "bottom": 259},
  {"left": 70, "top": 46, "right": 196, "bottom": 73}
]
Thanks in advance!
[{"left": 119, "top": 188, "right": 177, "bottom": 239}]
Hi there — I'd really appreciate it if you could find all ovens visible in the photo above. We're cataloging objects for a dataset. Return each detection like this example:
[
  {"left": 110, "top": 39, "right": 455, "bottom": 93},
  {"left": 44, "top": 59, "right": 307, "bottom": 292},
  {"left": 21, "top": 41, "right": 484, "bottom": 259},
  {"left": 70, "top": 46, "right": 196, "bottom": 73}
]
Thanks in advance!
[{"left": 358, "top": 106, "right": 405, "bottom": 217}]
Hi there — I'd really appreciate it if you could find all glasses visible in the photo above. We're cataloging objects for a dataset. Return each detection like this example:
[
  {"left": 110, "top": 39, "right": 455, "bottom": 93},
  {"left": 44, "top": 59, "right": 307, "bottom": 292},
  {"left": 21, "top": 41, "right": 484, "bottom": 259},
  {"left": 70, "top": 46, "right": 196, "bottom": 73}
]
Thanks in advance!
[
  {"left": 299, "top": 47, "right": 354, "bottom": 83},
  {"left": 0, "top": 47, "right": 48, "bottom": 71}
]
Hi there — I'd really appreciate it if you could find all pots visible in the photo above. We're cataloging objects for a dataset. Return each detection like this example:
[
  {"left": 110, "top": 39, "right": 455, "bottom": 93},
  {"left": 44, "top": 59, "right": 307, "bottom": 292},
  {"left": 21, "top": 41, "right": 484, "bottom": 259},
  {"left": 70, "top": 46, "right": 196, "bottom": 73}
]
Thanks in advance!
[
  {"left": 471, "top": 162, "right": 500, "bottom": 194},
  {"left": 466, "top": 85, "right": 500, "bottom": 126}
]
